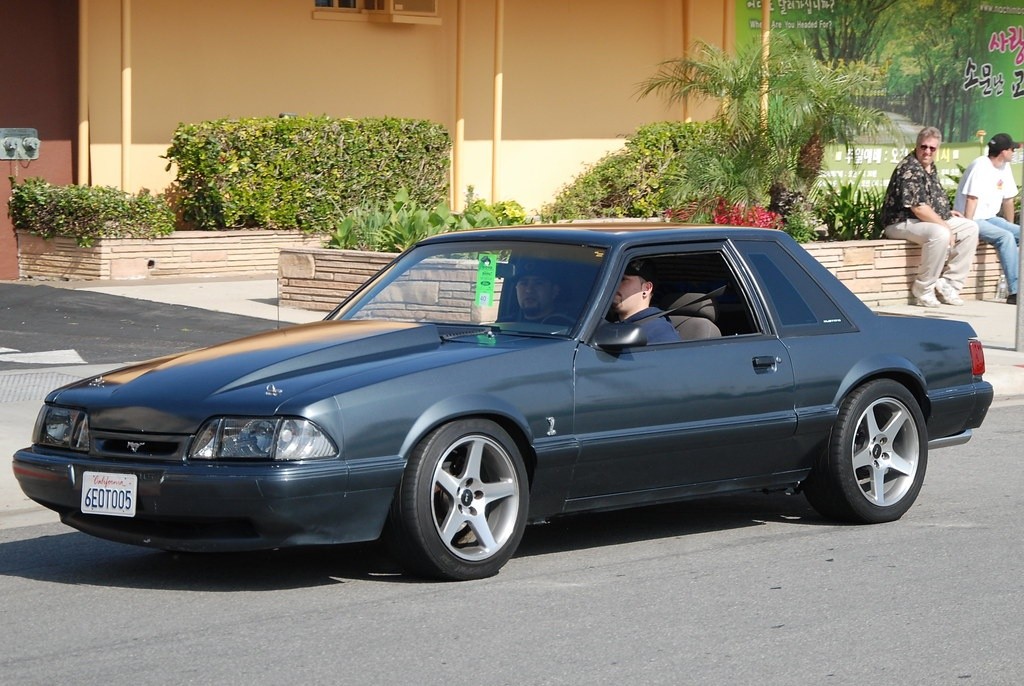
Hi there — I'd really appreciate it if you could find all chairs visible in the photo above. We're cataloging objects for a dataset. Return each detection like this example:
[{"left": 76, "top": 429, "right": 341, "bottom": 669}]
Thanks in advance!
[{"left": 664, "top": 290, "right": 722, "bottom": 342}]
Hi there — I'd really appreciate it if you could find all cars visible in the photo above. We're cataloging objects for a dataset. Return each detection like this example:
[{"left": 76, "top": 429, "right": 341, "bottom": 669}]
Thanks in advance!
[{"left": 13, "top": 220, "right": 995, "bottom": 581}]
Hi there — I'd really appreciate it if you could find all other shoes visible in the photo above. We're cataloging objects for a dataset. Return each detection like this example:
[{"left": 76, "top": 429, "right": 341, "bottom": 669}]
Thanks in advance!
[
  {"left": 935, "top": 281, "right": 965, "bottom": 306},
  {"left": 1006, "top": 294, "right": 1017, "bottom": 305},
  {"left": 912, "top": 286, "right": 941, "bottom": 307}
]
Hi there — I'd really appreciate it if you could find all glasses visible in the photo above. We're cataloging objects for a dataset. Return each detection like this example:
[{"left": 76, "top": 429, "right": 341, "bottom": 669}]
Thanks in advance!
[{"left": 917, "top": 145, "right": 937, "bottom": 152}]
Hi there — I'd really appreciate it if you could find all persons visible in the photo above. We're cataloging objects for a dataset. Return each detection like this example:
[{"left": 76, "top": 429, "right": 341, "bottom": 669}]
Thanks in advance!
[
  {"left": 879, "top": 126, "right": 978, "bottom": 308},
  {"left": 956, "top": 133, "right": 1020, "bottom": 306},
  {"left": 591, "top": 261, "right": 678, "bottom": 344},
  {"left": 492, "top": 258, "right": 566, "bottom": 328}
]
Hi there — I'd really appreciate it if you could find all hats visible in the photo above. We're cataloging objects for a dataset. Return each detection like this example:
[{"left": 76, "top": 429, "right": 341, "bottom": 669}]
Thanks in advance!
[
  {"left": 624, "top": 258, "right": 656, "bottom": 283},
  {"left": 514, "top": 258, "right": 557, "bottom": 285},
  {"left": 987, "top": 133, "right": 1020, "bottom": 153}
]
